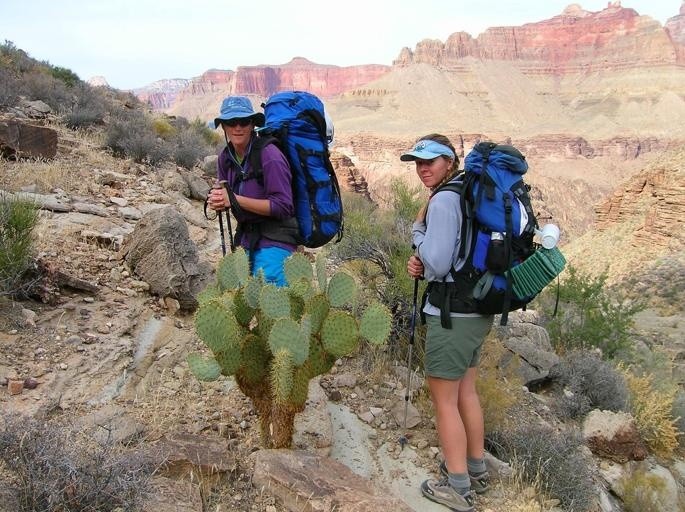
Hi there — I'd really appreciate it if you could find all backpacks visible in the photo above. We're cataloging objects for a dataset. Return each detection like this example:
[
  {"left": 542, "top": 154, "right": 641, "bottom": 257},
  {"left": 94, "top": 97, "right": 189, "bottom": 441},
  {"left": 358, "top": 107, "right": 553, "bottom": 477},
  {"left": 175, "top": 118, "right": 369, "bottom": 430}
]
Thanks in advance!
[
  {"left": 226, "top": 90, "right": 342, "bottom": 249},
  {"left": 424, "top": 142, "right": 540, "bottom": 314}
]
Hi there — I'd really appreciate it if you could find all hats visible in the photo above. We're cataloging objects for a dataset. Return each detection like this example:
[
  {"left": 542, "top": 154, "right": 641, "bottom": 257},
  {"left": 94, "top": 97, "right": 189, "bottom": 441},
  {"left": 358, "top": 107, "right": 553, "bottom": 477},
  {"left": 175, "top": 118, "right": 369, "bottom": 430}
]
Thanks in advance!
[
  {"left": 207, "top": 96, "right": 265, "bottom": 130},
  {"left": 400, "top": 139, "right": 456, "bottom": 161}
]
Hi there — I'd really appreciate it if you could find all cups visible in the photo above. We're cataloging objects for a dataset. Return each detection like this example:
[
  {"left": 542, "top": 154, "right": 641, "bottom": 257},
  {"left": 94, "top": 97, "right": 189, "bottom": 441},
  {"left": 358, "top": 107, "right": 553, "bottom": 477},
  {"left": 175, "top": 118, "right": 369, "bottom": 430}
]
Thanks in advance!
[{"left": 534, "top": 224, "right": 559, "bottom": 250}]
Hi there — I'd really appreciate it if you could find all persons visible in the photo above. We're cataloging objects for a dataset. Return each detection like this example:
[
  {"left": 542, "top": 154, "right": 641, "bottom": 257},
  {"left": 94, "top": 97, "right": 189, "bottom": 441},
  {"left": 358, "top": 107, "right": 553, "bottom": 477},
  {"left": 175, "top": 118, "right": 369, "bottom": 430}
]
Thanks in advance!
[
  {"left": 397, "top": 134, "right": 498, "bottom": 511},
  {"left": 204, "top": 97, "right": 298, "bottom": 290}
]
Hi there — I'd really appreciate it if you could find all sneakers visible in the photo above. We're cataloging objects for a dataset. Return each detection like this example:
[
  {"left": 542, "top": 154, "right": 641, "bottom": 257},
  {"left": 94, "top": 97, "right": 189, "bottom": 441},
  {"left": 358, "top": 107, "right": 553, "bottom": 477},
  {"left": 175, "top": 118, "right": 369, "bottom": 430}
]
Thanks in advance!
[
  {"left": 420, "top": 479, "right": 477, "bottom": 511},
  {"left": 440, "top": 460, "right": 490, "bottom": 493}
]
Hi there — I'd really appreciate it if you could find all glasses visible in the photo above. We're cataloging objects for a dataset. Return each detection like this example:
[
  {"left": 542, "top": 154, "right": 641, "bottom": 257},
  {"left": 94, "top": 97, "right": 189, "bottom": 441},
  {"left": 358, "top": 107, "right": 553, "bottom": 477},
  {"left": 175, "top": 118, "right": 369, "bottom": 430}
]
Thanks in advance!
[{"left": 221, "top": 118, "right": 253, "bottom": 128}]
